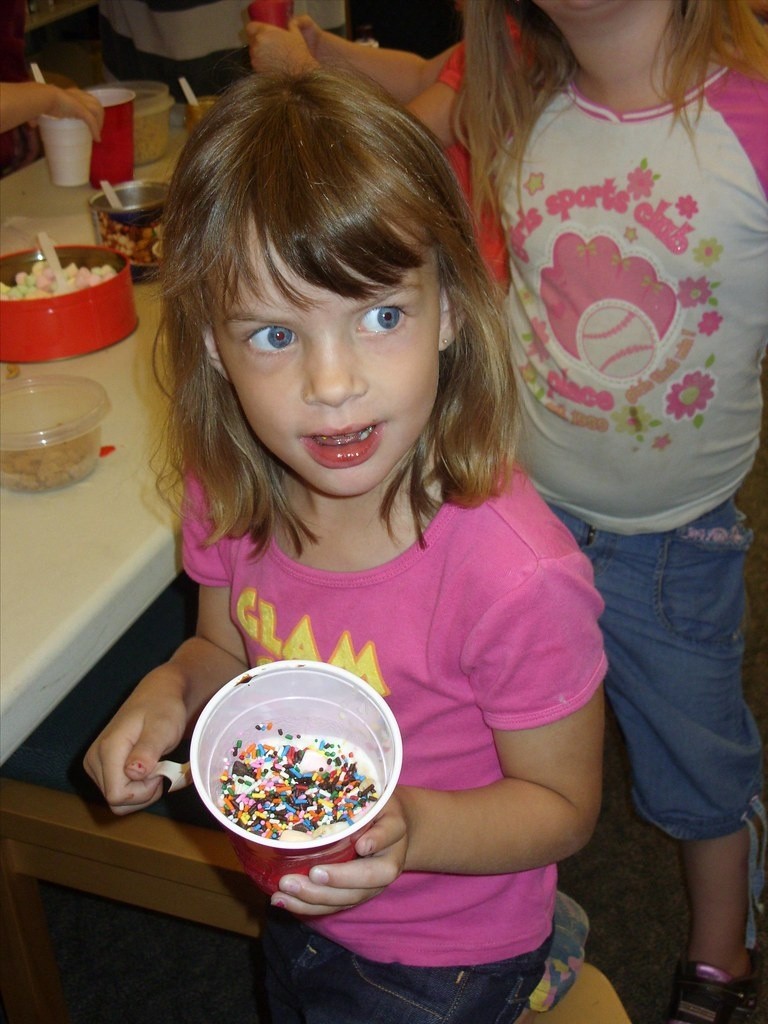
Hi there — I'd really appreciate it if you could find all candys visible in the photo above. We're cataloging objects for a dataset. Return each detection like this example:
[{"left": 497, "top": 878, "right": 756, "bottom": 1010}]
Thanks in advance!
[{"left": 0, "top": 260, "right": 119, "bottom": 301}]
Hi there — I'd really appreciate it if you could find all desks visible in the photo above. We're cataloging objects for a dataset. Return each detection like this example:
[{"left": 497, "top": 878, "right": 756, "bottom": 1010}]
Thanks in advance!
[{"left": 0, "top": 102, "right": 470, "bottom": 1024}]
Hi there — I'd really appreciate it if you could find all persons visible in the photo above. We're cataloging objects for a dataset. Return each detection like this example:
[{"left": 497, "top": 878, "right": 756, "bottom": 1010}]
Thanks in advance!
[
  {"left": 84, "top": 68, "right": 611, "bottom": 1024},
  {"left": 100, "top": 0, "right": 347, "bottom": 97},
  {"left": 452, "top": 0, "right": 768, "bottom": 1024},
  {"left": 0, "top": 81, "right": 103, "bottom": 143},
  {"left": 247, "top": 0, "right": 533, "bottom": 296}
]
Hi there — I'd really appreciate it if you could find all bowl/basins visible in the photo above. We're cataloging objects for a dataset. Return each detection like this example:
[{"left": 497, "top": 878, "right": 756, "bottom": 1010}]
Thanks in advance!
[
  {"left": 0, "top": 375, "right": 110, "bottom": 490},
  {"left": 0, "top": 245, "right": 138, "bottom": 362},
  {"left": 96, "top": 81, "right": 174, "bottom": 165}
]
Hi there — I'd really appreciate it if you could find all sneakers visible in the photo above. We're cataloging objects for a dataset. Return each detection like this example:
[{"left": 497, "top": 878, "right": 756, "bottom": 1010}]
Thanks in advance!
[{"left": 666, "top": 941, "right": 762, "bottom": 1024}]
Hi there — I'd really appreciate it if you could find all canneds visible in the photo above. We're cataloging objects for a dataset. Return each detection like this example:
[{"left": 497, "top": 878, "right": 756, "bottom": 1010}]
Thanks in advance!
[{"left": 88, "top": 180, "right": 169, "bottom": 284}]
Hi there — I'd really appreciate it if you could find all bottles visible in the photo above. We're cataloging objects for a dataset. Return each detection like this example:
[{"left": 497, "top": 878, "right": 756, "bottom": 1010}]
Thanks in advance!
[{"left": 354, "top": 21, "right": 381, "bottom": 52}]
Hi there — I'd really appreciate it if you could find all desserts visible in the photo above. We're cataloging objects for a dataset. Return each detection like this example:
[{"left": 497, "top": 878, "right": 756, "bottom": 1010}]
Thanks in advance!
[{"left": 218, "top": 721, "right": 379, "bottom": 845}]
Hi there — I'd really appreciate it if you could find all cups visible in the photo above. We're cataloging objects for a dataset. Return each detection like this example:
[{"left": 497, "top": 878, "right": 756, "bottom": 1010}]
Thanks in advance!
[
  {"left": 247, "top": 1, "right": 294, "bottom": 30},
  {"left": 187, "top": 95, "right": 221, "bottom": 134},
  {"left": 38, "top": 113, "right": 92, "bottom": 188},
  {"left": 192, "top": 661, "right": 404, "bottom": 895},
  {"left": 84, "top": 88, "right": 135, "bottom": 190}
]
